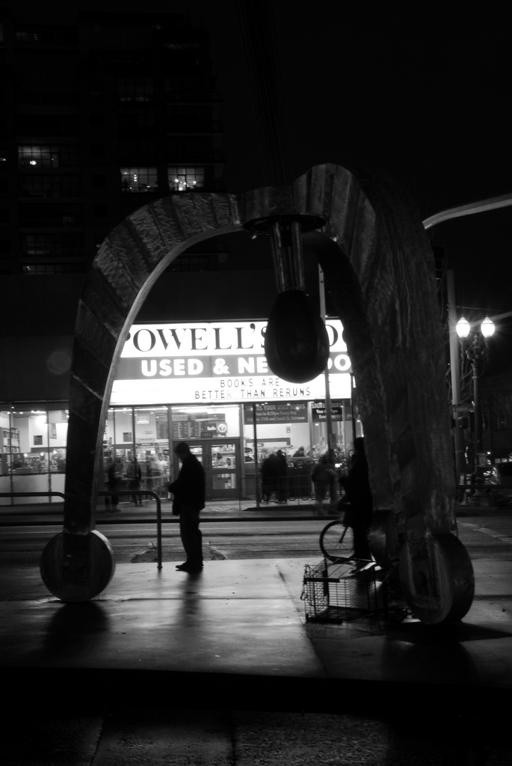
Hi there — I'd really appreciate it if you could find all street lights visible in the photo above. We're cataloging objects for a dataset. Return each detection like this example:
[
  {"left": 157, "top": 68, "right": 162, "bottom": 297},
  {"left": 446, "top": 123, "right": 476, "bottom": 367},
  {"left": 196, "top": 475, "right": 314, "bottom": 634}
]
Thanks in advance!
[{"left": 454, "top": 312, "right": 496, "bottom": 477}]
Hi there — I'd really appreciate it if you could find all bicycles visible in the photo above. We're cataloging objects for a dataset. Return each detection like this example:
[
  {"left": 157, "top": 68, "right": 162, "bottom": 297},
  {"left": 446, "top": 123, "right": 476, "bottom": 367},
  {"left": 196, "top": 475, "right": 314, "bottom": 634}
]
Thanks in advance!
[{"left": 319, "top": 503, "right": 358, "bottom": 564}]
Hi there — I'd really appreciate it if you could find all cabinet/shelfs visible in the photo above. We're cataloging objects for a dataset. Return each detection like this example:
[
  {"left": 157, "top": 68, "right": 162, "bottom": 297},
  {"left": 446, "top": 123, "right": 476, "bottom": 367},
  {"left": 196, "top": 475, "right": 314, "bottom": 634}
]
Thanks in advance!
[
  {"left": 0, "top": 428, "right": 21, "bottom": 473},
  {"left": 104, "top": 441, "right": 237, "bottom": 502}
]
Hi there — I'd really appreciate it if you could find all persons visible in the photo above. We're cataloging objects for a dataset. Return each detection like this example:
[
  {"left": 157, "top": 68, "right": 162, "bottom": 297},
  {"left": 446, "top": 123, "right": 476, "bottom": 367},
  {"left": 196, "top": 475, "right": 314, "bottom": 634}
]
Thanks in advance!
[
  {"left": 126, "top": 457, "right": 142, "bottom": 506},
  {"left": 163, "top": 442, "right": 207, "bottom": 573},
  {"left": 214, "top": 453, "right": 225, "bottom": 466},
  {"left": 341, "top": 438, "right": 374, "bottom": 573},
  {"left": 261, "top": 446, "right": 334, "bottom": 502},
  {"left": 226, "top": 458, "right": 231, "bottom": 465},
  {"left": 105, "top": 463, "right": 121, "bottom": 512}
]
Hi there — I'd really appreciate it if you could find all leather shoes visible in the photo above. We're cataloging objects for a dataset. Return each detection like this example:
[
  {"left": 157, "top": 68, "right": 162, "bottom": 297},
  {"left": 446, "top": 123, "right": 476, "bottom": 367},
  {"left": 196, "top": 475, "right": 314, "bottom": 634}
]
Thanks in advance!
[{"left": 176, "top": 561, "right": 203, "bottom": 571}]
